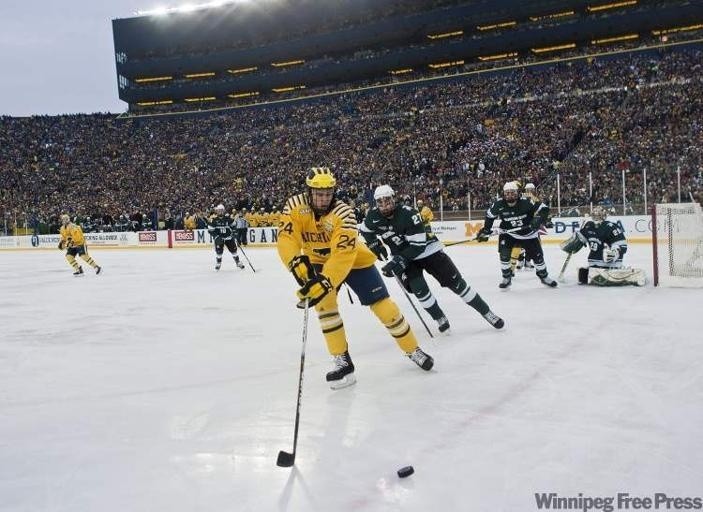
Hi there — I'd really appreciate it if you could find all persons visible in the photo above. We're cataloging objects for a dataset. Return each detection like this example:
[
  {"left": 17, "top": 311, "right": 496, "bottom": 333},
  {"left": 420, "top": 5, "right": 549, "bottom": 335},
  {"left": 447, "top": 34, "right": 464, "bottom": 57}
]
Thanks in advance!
[
  {"left": 359, "top": 185, "right": 506, "bottom": 335},
  {"left": 247, "top": 206, "right": 258, "bottom": 227},
  {"left": 560, "top": 204, "right": 628, "bottom": 287},
  {"left": 1, "top": 114, "right": 57, "bottom": 237},
  {"left": 257, "top": 208, "right": 269, "bottom": 228},
  {"left": 475, "top": 180, "right": 556, "bottom": 288},
  {"left": 58, "top": 213, "right": 102, "bottom": 276},
  {"left": 277, "top": 111, "right": 701, "bottom": 186},
  {"left": 208, "top": 111, "right": 278, "bottom": 205},
  {"left": 268, "top": 206, "right": 282, "bottom": 227},
  {"left": 278, "top": 186, "right": 703, "bottom": 212},
  {"left": 231, "top": 213, "right": 249, "bottom": 245},
  {"left": 137, "top": 2, "right": 703, "bottom": 110},
  {"left": 277, "top": 167, "right": 434, "bottom": 391},
  {"left": 228, "top": 208, "right": 239, "bottom": 228},
  {"left": 239, "top": 207, "right": 250, "bottom": 229},
  {"left": 58, "top": 112, "right": 207, "bottom": 231},
  {"left": 206, "top": 204, "right": 247, "bottom": 272}
]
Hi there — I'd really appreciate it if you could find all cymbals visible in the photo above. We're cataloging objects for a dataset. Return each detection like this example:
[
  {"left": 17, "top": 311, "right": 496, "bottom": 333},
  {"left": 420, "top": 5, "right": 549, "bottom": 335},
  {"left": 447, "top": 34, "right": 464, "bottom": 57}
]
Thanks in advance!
[{"left": 276, "top": 277, "right": 309, "bottom": 468}]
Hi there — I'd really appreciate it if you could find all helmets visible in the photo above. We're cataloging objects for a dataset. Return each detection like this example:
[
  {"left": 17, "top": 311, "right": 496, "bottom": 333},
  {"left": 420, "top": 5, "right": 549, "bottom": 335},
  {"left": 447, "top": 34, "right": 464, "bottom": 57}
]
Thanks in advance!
[
  {"left": 591, "top": 206, "right": 606, "bottom": 225},
  {"left": 306, "top": 167, "right": 336, "bottom": 213},
  {"left": 61, "top": 214, "right": 70, "bottom": 225},
  {"left": 374, "top": 184, "right": 396, "bottom": 216},
  {"left": 185, "top": 211, "right": 189, "bottom": 214},
  {"left": 418, "top": 200, "right": 423, "bottom": 209},
  {"left": 216, "top": 205, "right": 226, "bottom": 217},
  {"left": 231, "top": 205, "right": 277, "bottom": 214},
  {"left": 503, "top": 182, "right": 519, "bottom": 202}
]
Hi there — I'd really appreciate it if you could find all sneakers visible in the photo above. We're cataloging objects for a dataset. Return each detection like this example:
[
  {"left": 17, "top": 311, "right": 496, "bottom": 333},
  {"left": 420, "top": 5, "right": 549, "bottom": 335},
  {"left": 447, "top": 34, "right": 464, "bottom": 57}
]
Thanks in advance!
[
  {"left": 406, "top": 346, "right": 433, "bottom": 371},
  {"left": 94, "top": 265, "right": 100, "bottom": 274},
  {"left": 541, "top": 277, "right": 557, "bottom": 287},
  {"left": 484, "top": 311, "right": 504, "bottom": 329},
  {"left": 437, "top": 316, "right": 449, "bottom": 333},
  {"left": 522, "top": 261, "right": 533, "bottom": 267},
  {"left": 326, "top": 352, "right": 355, "bottom": 381},
  {"left": 516, "top": 261, "right": 524, "bottom": 270},
  {"left": 73, "top": 265, "right": 83, "bottom": 274},
  {"left": 499, "top": 277, "right": 511, "bottom": 288},
  {"left": 215, "top": 261, "right": 223, "bottom": 269},
  {"left": 236, "top": 260, "right": 244, "bottom": 268}
]
